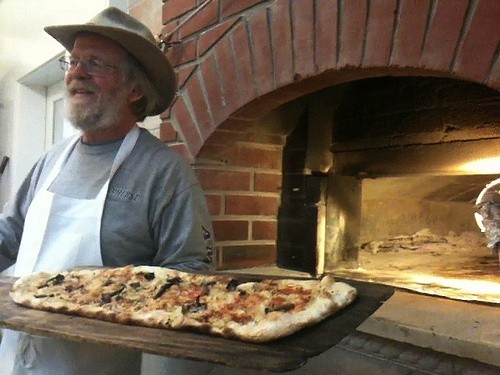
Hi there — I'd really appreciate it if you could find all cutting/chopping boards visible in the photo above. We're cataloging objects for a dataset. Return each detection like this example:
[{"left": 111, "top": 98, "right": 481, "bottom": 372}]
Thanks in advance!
[{"left": 1, "top": 266, "right": 395, "bottom": 372}]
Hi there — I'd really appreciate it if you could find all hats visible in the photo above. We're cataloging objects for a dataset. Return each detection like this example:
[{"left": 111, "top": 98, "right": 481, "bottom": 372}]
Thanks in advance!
[{"left": 44, "top": 7, "right": 175, "bottom": 116}]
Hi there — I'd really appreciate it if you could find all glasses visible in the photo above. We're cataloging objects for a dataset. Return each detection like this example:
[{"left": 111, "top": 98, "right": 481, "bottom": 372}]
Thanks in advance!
[{"left": 59, "top": 54, "right": 133, "bottom": 77}]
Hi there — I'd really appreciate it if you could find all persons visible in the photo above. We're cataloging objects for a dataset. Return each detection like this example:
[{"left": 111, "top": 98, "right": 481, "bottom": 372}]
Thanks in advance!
[{"left": 1, "top": 8, "right": 207, "bottom": 375}]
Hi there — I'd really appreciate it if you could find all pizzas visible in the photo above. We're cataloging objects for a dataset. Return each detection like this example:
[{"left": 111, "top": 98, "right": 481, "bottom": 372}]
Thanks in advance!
[{"left": 8, "top": 265, "right": 357, "bottom": 343}]
